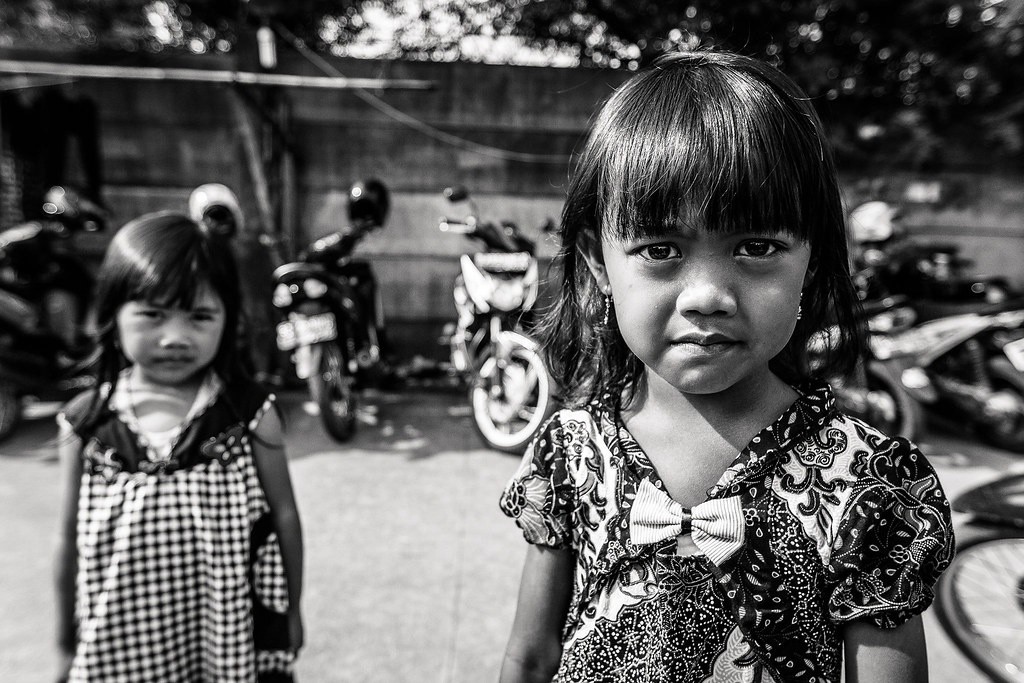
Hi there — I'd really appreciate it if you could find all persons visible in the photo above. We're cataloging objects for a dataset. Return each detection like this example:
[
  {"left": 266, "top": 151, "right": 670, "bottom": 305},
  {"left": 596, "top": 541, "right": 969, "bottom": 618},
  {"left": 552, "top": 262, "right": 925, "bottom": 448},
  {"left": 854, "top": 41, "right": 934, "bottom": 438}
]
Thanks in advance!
[
  {"left": 495, "top": 50, "right": 955, "bottom": 683},
  {"left": 52, "top": 208, "right": 302, "bottom": 683}
]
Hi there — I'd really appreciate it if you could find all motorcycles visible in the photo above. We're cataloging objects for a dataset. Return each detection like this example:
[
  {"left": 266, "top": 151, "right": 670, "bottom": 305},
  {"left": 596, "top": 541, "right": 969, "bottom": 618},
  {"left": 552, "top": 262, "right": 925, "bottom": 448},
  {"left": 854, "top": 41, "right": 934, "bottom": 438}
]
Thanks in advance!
[
  {"left": 0, "top": 187, "right": 110, "bottom": 448},
  {"left": 252, "top": 202, "right": 385, "bottom": 442},
  {"left": 935, "top": 475, "right": 1024, "bottom": 683},
  {"left": 812, "top": 175, "right": 1022, "bottom": 458},
  {"left": 436, "top": 181, "right": 564, "bottom": 458}
]
